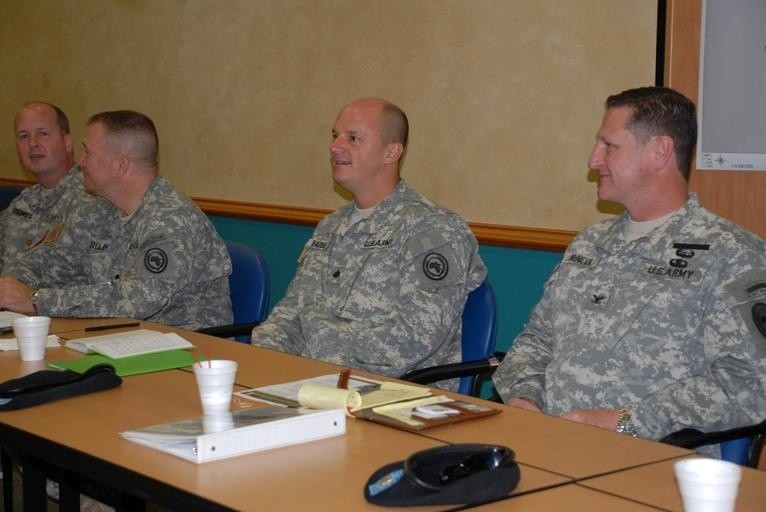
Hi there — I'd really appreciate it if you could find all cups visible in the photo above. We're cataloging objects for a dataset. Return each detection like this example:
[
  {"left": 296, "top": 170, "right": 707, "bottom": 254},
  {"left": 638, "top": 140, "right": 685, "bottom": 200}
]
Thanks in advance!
[
  {"left": 191, "top": 359, "right": 238, "bottom": 416},
  {"left": 11, "top": 316, "right": 52, "bottom": 362},
  {"left": 672, "top": 457, "right": 742, "bottom": 512}
]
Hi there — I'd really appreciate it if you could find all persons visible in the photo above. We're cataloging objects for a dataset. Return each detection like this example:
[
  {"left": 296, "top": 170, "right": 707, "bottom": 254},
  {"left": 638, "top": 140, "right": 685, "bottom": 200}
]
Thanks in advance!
[
  {"left": 0, "top": 101, "right": 121, "bottom": 282},
  {"left": 0, "top": 110, "right": 236, "bottom": 342},
  {"left": 251, "top": 97, "right": 488, "bottom": 393},
  {"left": 491, "top": 86, "right": 766, "bottom": 460}
]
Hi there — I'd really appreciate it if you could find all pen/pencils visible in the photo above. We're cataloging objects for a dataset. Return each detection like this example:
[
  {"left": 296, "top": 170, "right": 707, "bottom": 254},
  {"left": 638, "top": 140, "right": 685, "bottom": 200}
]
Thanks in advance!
[{"left": 85, "top": 322, "right": 139, "bottom": 331}]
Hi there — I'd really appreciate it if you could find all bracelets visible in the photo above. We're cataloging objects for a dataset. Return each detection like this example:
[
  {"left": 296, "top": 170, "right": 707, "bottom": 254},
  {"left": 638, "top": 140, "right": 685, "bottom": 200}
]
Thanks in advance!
[
  {"left": 616, "top": 406, "right": 636, "bottom": 437},
  {"left": 32, "top": 290, "right": 40, "bottom": 315}
]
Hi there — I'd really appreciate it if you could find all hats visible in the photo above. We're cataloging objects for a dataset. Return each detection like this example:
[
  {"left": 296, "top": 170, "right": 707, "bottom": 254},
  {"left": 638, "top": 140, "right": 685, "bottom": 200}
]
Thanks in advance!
[{"left": 364, "top": 442, "right": 520, "bottom": 506}]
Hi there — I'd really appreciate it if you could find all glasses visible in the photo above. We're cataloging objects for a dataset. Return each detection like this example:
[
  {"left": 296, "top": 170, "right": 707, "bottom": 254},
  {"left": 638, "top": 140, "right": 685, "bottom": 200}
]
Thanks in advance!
[{"left": 439, "top": 448, "right": 504, "bottom": 485}]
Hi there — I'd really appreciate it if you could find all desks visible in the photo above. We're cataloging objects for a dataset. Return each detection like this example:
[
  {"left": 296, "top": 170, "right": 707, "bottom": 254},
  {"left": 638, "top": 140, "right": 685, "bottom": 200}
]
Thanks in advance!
[{"left": 1, "top": 317, "right": 765, "bottom": 511}]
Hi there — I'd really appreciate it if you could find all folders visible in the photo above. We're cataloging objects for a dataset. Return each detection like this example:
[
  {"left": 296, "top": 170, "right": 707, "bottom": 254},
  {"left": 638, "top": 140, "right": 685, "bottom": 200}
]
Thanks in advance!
[{"left": 119, "top": 406, "right": 346, "bottom": 465}]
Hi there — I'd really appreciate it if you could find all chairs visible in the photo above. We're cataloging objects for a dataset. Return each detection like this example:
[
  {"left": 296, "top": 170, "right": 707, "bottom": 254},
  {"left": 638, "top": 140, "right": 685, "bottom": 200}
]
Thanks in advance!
[
  {"left": 399, "top": 280, "right": 507, "bottom": 396},
  {"left": 193, "top": 241, "right": 271, "bottom": 345}
]
